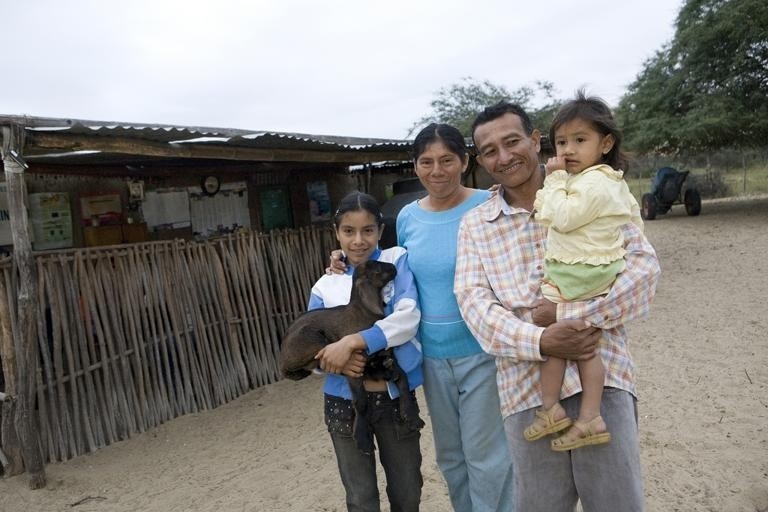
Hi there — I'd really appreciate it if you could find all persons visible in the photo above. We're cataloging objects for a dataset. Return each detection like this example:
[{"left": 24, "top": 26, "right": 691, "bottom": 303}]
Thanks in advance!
[
  {"left": 453, "top": 100, "right": 662, "bottom": 510},
  {"left": 325, "top": 123, "right": 519, "bottom": 511},
  {"left": 522, "top": 90, "right": 646, "bottom": 451},
  {"left": 308, "top": 191, "right": 426, "bottom": 512}
]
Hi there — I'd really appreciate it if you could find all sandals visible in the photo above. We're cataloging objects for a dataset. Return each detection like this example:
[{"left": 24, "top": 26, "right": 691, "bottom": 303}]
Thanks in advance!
[
  {"left": 550, "top": 414, "right": 612, "bottom": 451},
  {"left": 523, "top": 401, "right": 573, "bottom": 442}
]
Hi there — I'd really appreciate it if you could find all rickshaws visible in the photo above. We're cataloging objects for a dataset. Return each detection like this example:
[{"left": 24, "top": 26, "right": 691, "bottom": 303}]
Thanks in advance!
[{"left": 642, "top": 166, "right": 701, "bottom": 221}]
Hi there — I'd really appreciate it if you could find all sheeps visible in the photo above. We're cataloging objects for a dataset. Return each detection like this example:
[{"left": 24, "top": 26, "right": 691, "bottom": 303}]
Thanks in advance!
[{"left": 277, "top": 260, "right": 425, "bottom": 454}]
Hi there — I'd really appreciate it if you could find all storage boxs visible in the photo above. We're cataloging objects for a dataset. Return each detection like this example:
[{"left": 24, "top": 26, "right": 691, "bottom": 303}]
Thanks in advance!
[
  {"left": 28, "top": 191, "right": 74, "bottom": 252},
  {"left": 76, "top": 191, "right": 147, "bottom": 248}
]
